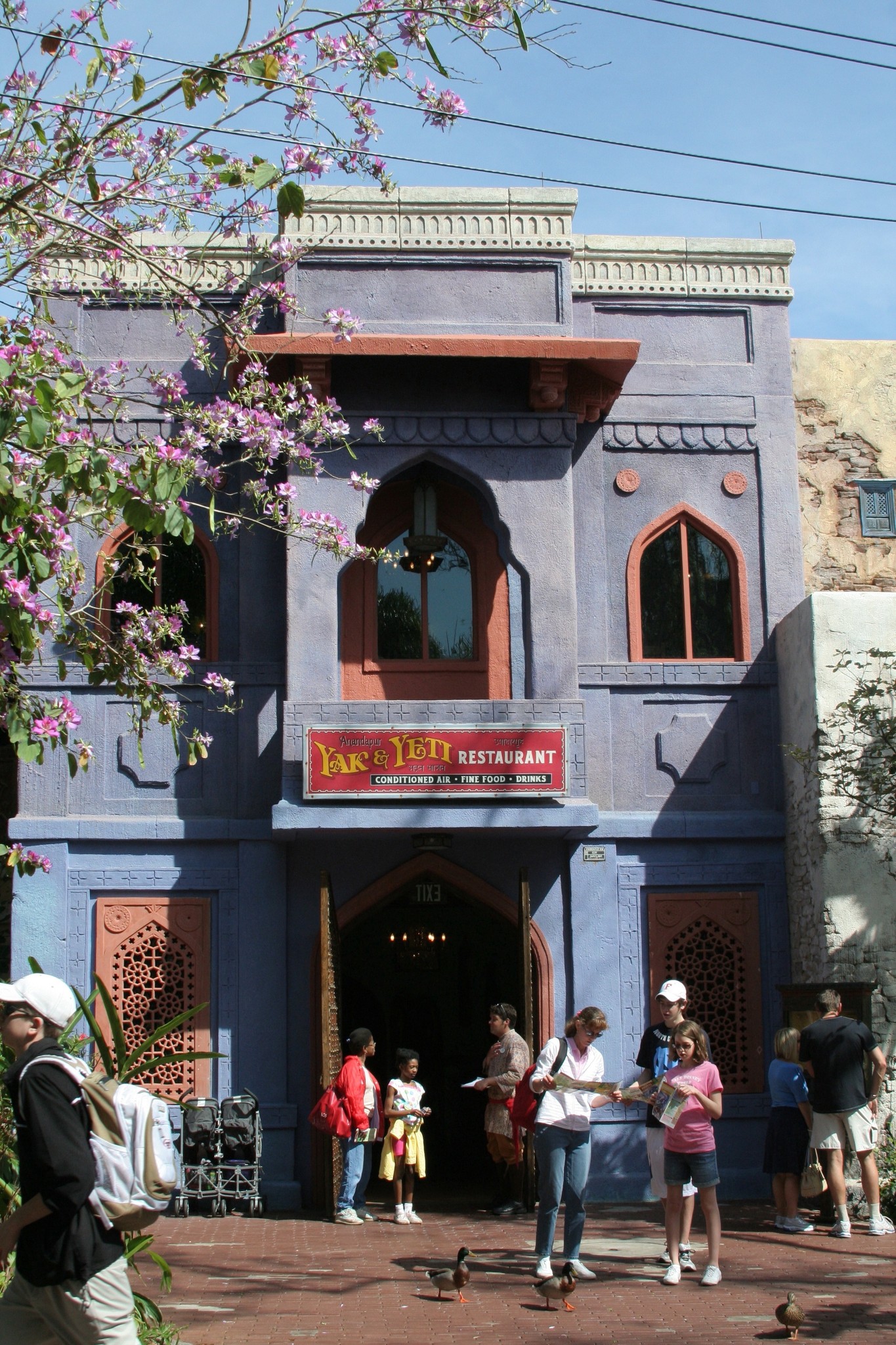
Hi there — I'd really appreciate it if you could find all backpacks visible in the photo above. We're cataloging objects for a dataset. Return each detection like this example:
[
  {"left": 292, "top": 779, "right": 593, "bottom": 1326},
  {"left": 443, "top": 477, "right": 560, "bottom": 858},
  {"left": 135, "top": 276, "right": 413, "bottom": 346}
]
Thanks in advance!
[{"left": 18, "top": 1051, "right": 178, "bottom": 1232}]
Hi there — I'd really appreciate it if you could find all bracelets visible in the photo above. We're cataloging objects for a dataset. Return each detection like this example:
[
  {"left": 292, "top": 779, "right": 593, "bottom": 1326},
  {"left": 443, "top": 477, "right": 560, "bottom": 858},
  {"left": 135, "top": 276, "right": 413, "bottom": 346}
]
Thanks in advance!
[
  {"left": 869, "top": 1093, "right": 878, "bottom": 1099},
  {"left": 807, "top": 1129, "right": 814, "bottom": 1132},
  {"left": 540, "top": 1079, "right": 542, "bottom": 1083}
]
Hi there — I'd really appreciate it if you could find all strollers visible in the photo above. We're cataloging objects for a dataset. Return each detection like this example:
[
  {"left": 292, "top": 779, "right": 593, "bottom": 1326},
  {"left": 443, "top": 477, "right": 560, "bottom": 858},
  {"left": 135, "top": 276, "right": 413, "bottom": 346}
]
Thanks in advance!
[{"left": 172, "top": 1086, "right": 265, "bottom": 1218}]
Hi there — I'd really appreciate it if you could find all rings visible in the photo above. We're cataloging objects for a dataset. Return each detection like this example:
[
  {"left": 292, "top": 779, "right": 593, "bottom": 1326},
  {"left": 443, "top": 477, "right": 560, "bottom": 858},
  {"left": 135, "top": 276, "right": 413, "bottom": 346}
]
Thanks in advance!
[{"left": 494, "top": 1049, "right": 497, "bottom": 1053}]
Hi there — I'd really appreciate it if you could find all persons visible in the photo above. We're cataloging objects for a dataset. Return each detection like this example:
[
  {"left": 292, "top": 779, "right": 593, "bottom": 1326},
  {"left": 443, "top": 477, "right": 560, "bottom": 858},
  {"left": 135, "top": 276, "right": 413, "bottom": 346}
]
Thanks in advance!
[
  {"left": 648, "top": 1020, "right": 723, "bottom": 1288},
  {"left": 0, "top": 974, "right": 141, "bottom": 1345},
  {"left": 334, "top": 1028, "right": 385, "bottom": 1226},
  {"left": 762, "top": 1027, "right": 817, "bottom": 1232},
  {"left": 798, "top": 989, "right": 896, "bottom": 1238},
  {"left": 473, "top": 1003, "right": 530, "bottom": 1215},
  {"left": 378, "top": 1048, "right": 432, "bottom": 1224},
  {"left": 529, "top": 1006, "right": 622, "bottom": 1280},
  {"left": 622, "top": 979, "right": 713, "bottom": 1272}
]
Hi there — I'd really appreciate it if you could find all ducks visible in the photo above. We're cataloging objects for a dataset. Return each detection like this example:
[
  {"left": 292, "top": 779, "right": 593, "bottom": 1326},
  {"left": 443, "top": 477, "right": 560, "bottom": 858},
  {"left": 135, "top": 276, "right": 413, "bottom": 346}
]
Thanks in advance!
[
  {"left": 775, "top": 1292, "right": 806, "bottom": 1341},
  {"left": 531, "top": 1262, "right": 579, "bottom": 1311},
  {"left": 425, "top": 1247, "right": 477, "bottom": 1302}
]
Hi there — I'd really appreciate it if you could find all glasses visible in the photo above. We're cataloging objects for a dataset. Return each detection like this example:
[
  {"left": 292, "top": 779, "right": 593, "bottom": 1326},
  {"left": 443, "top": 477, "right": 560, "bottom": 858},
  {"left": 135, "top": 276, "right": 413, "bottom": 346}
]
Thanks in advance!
[
  {"left": 675, "top": 1043, "right": 694, "bottom": 1050},
  {"left": 4, "top": 1003, "right": 52, "bottom": 1027},
  {"left": 583, "top": 1026, "right": 603, "bottom": 1038},
  {"left": 368, "top": 1041, "right": 376, "bottom": 1047},
  {"left": 497, "top": 1002, "right": 507, "bottom": 1017}
]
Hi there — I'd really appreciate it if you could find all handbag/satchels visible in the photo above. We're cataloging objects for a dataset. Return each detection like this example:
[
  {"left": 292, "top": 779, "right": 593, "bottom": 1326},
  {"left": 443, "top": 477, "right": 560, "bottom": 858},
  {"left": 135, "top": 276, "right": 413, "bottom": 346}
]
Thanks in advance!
[
  {"left": 800, "top": 1140, "right": 828, "bottom": 1198},
  {"left": 509, "top": 1036, "right": 568, "bottom": 1131}
]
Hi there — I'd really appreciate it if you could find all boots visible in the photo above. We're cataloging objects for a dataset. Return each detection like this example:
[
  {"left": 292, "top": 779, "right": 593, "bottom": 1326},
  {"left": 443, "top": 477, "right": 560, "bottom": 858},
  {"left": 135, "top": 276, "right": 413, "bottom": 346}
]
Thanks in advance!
[
  {"left": 477, "top": 1162, "right": 511, "bottom": 1211},
  {"left": 493, "top": 1160, "right": 527, "bottom": 1215}
]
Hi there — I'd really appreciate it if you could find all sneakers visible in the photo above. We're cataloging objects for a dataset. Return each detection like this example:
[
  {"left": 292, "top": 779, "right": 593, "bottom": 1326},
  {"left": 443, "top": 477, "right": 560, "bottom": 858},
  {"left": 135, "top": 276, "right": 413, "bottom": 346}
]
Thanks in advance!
[
  {"left": 308, "top": 1086, "right": 353, "bottom": 1138},
  {"left": 829, "top": 1220, "right": 852, "bottom": 1238},
  {"left": 355, "top": 1207, "right": 379, "bottom": 1222},
  {"left": 774, "top": 1215, "right": 785, "bottom": 1228},
  {"left": 700, "top": 1264, "right": 722, "bottom": 1286},
  {"left": 393, "top": 1210, "right": 410, "bottom": 1225},
  {"left": 405, "top": 1210, "right": 423, "bottom": 1224},
  {"left": 868, "top": 1213, "right": 896, "bottom": 1235},
  {"left": 334, "top": 1208, "right": 364, "bottom": 1225},
  {"left": 659, "top": 1239, "right": 690, "bottom": 1263},
  {"left": 535, "top": 1259, "right": 553, "bottom": 1279},
  {"left": 679, "top": 1244, "right": 697, "bottom": 1272},
  {"left": 566, "top": 1259, "right": 597, "bottom": 1280},
  {"left": 663, "top": 1259, "right": 681, "bottom": 1285},
  {"left": 782, "top": 1214, "right": 816, "bottom": 1231}
]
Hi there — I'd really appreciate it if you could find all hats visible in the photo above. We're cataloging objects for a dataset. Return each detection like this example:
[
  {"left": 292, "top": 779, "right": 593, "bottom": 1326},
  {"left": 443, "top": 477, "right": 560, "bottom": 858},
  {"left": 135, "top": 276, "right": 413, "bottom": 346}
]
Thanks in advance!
[
  {"left": 655, "top": 979, "right": 686, "bottom": 1002},
  {"left": 0, "top": 973, "right": 77, "bottom": 1028}
]
